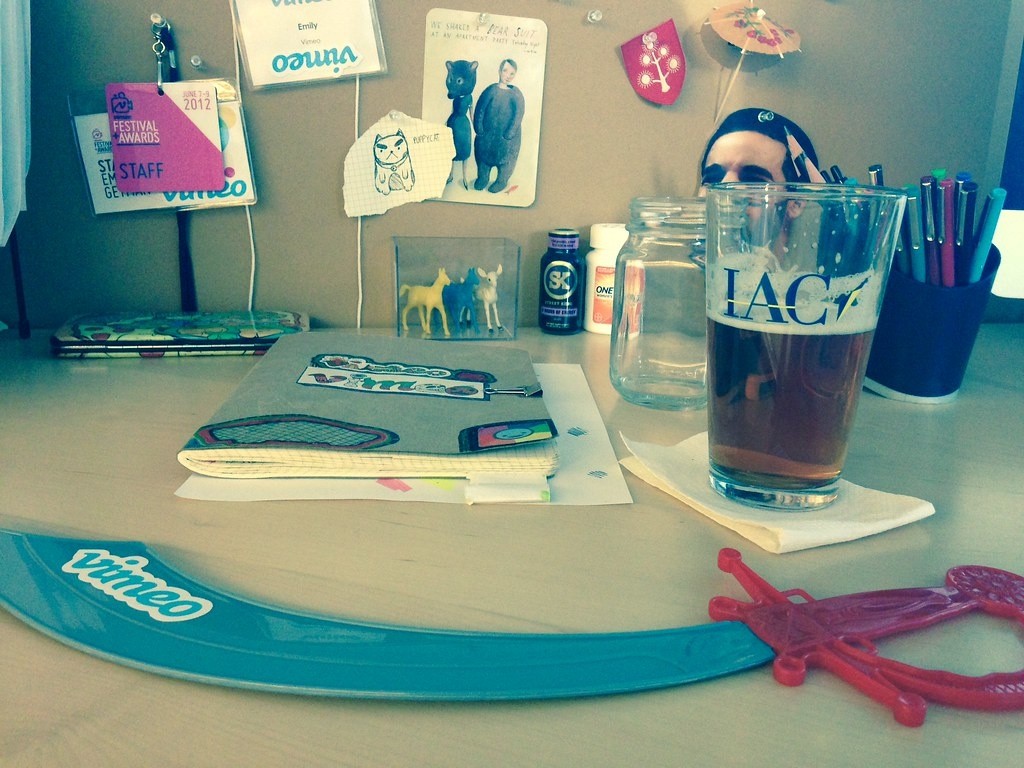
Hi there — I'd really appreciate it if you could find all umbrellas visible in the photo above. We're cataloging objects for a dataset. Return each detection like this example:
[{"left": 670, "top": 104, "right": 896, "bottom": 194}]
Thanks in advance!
[{"left": 703, "top": 0, "right": 801, "bottom": 125}]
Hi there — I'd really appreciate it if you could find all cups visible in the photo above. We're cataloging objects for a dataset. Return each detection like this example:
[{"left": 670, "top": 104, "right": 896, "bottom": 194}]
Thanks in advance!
[
  {"left": 704, "top": 180, "right": 909, "bottom": 514},
  {"left": 860, "top": 240, "right": 1001, "bottom": 405}
]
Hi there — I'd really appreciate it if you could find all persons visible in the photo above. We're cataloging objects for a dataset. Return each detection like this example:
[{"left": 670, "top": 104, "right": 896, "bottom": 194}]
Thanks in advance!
[{"left": 693, "top": 106, "right": 820, "bottom": 266}]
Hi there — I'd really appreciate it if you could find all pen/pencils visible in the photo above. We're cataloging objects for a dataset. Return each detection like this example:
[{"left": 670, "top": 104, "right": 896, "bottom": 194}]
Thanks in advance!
[{"left": 781, "top": 123, "right": 1008, "bottom": 288}]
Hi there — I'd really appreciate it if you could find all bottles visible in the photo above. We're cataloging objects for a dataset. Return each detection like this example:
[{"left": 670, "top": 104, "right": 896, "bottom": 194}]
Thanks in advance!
[
  {"left": 605, "top": 197, "right": 708, "bottom": 410},
  {"left": 537, "top": 223, "right": 647, "bottom": 337}
]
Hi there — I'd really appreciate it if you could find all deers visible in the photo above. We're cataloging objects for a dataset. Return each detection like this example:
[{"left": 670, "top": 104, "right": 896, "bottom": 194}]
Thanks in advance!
[{"left": 459, "top": 264, "right": 503, "bottom": 333}]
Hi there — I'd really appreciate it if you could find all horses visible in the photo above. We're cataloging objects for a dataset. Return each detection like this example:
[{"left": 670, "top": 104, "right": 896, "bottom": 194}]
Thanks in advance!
[{"left": 399, "top": 267, "right": 481, "bottom": 336}]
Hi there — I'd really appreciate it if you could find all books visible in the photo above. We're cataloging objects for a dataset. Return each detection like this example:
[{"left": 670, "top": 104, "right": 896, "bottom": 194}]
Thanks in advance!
[{"left": 176, "top": 332, "right": 563, "bottom": 481}]
